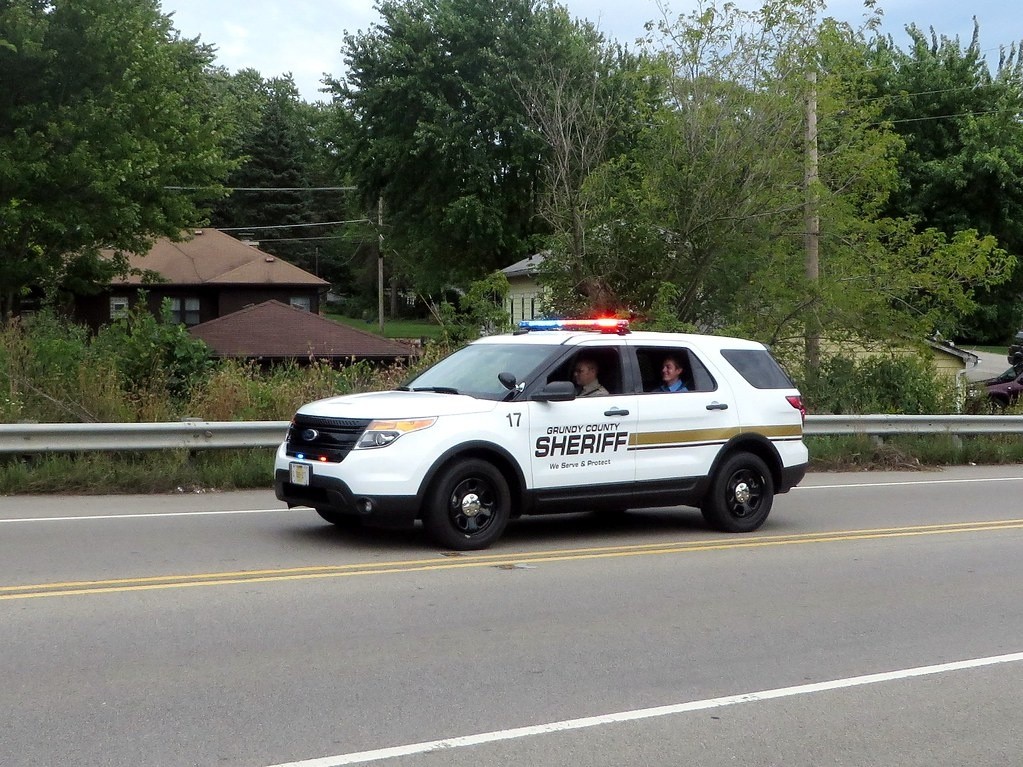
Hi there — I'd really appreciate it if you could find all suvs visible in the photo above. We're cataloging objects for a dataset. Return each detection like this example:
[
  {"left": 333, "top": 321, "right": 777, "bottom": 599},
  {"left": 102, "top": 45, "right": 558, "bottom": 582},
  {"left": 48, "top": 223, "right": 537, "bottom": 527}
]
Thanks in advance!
[{"left": 271, "top": 330, "right": 811, "bottom": 553}]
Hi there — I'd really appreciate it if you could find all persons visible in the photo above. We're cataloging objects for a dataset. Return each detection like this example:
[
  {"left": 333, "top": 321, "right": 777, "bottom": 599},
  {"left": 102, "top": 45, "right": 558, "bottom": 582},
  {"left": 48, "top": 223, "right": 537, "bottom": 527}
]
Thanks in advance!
[
  {"left": 652, "top": 355, "right": 689, "bottom": 392},
  {"left": 573, "top": 356, "right": 608, "bottom": 396}
]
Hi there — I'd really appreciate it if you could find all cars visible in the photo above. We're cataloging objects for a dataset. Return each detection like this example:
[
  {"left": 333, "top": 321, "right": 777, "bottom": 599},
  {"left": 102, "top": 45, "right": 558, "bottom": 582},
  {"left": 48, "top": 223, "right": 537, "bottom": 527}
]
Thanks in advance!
[{"left": 966, "top": 365, "right": 1023, "bottom": 410}]
